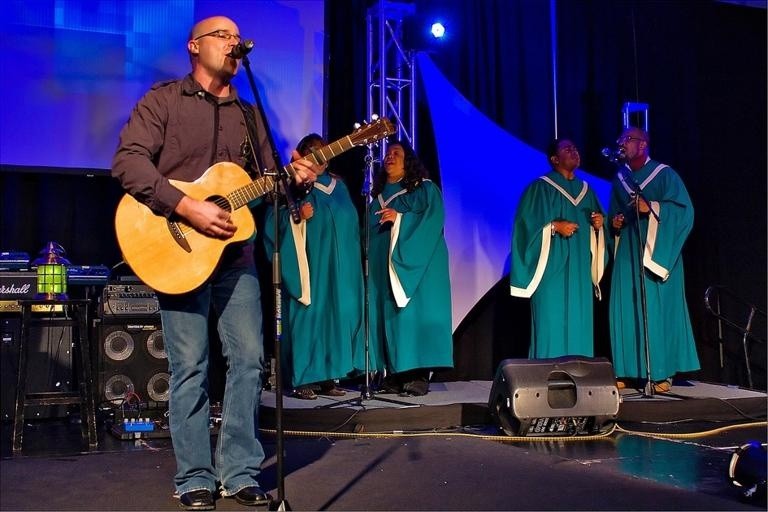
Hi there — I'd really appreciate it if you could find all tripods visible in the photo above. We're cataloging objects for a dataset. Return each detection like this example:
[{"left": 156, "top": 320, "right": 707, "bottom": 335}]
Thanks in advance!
[
  {"left": 314, "top": 169, "right": 424, "bottom": 409},
  {"left": 621, "top": 195, "right": 699, "bottom": 402}
]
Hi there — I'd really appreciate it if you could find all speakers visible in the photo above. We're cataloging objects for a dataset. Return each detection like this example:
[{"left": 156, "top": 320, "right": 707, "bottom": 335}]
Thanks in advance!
[
  {"left": 2, "top": 274, "right": 78, "bottom": 406},
  {"left": 96, "top": 321, "right": 172, "bottom": 413}
]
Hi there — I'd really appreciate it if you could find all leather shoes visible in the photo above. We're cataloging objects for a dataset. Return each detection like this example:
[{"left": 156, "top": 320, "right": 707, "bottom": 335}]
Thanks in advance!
[
  {"left": 179, "top": 490, "right": 216, "bottom": 511},
  {"left": 399, "top": 391, "right": 420, "bottom": 397},
  {"left": 233, "top": 486, "right": 275, "bottom": 506},
  {"left": 372, "top": 386, "right": 390, "bottom": 396}
]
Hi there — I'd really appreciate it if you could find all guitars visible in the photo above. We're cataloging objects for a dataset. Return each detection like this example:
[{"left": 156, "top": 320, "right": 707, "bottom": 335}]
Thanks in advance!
[{"left": 115, "top": 112, "right": 396, "bottom": 296}]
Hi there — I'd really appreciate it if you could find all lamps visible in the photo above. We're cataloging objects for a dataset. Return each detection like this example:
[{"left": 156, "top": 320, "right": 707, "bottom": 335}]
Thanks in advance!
[{"left": 38, "top": 263, "right": 67, "bottom": 298}]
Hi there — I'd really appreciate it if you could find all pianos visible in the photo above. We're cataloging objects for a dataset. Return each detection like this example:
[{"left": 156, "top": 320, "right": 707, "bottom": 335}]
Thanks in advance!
[{"left": 0, "top": 264, "right": 110, "bottom": 285}]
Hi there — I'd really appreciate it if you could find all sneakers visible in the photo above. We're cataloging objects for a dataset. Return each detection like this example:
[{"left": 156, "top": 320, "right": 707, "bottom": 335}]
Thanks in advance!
[
  {"left": 655, "top": 381, "right": 674, "bottom": 394},
  {"left": 287, "top": 390, "right": 318, "bottom": 401},
  {"left": 617, "top": 379, "right": 636, "bottom": 390},
  {"left": 322, "top": 388, "right": 348, "bottom": 396}
]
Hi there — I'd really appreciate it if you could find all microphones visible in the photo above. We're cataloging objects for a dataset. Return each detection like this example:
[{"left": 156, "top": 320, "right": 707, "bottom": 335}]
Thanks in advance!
[
  {"left": 602, "top": 148, "right": 625, "bottom": 160},
  {"left": 231, "top": 40, "right": 254, "bottom": 59}
]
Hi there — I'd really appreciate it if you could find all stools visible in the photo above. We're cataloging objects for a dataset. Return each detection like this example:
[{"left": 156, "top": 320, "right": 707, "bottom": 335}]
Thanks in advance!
[{"left": 12, "top": 296, "right": 96, "bottom": 452}]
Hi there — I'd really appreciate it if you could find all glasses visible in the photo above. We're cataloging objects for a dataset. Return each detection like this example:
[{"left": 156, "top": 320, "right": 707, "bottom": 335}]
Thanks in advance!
[
  {"left": 194, "top": 29, "right": 242, "bottom": 42},
  {"left": 615, "top": 133, "right": 648, "bottom": 144}
]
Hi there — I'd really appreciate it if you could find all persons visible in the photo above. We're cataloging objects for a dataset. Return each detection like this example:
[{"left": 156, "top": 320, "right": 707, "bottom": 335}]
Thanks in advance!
[
  {"left": 509, "top": 137, "right": 609, "bottom": 360},
  {"left": 608, "top": 127, "right": 702, "bottom": 396},
  {"left": 264, "top": 133, "right": 364, "bottom": 400},
  {"left": 111, "top": 15, "right": 326, "bottom": 511},
  {"left": 363, "top": 141, "right": 454, "bottom": 396}
]
